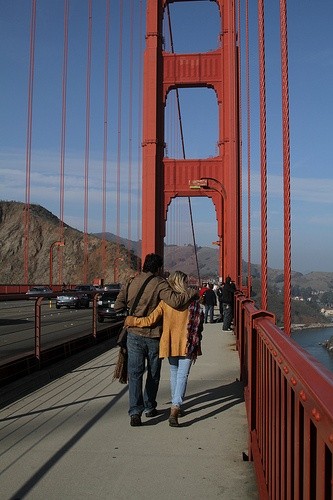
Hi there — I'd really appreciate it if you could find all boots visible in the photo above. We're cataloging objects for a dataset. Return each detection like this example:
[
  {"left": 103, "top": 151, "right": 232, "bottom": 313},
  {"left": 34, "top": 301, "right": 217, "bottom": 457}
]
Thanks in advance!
[{"left": 168, "top": 406, "right": 184, "bottom": 427}]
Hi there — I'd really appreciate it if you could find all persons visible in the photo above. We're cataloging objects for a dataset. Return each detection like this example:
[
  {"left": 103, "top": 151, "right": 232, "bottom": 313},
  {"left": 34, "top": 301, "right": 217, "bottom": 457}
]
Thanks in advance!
[
  {"left": 113, "top": 253, "right": 198, "bottom": 426},
  {"left": 199, "top": 277, "right": 236, "bottom": 333},
  {"left": 122, "top": 271, "right": 202, "bottom": 426}
]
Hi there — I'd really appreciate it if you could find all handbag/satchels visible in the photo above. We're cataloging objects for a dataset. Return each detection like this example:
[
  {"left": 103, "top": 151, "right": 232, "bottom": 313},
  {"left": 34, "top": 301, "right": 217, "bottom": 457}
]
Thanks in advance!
[{"left": 116, "top": 326, "right": 127, "bottom": 348}]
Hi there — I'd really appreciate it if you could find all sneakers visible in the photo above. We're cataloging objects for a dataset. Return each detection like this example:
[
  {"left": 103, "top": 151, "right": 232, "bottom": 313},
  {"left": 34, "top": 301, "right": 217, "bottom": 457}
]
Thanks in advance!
[
  {"left": 222, "top": 328, "right": 232, "bottom": 332},
  {"left": 146, "top": 409, "right": 161, "bottom": 417},
  {"left": 130, "top": 414, "right": 143, "bottom": 426}
]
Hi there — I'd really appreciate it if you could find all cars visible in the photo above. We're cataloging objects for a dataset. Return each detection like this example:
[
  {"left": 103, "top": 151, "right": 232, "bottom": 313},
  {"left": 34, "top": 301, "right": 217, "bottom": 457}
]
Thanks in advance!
[
  {"left": 96, "top": 288, "right": 126, "bottom": 322},
  {"left": 55, "top": 289, "right": 89, "bottom": 309},
  {"left": 26, "top": 287, "right": 53, "bottom": 302}
]
